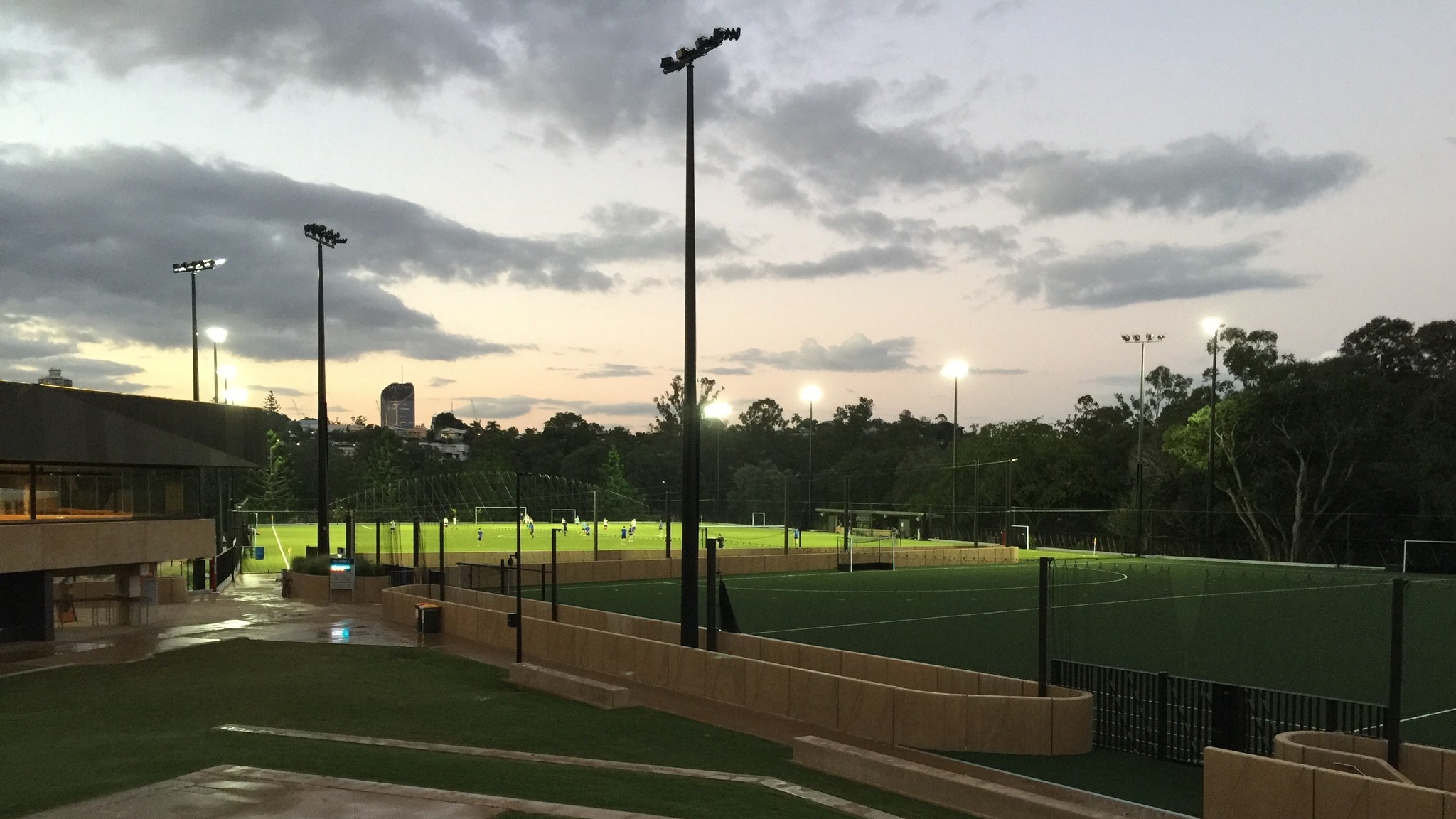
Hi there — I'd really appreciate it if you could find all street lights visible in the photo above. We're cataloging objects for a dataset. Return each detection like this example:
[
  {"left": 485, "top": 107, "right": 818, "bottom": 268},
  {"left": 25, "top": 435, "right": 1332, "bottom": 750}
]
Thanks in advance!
[
  {"left": 515, "top": 470, "right": 534, "bottom": 662},
  {"left": 171, "top": 258, "right": 216, "bottom": 400},
  {"left": 1206, "top": 321, "right": 1226, "bottom": 558},
  {"left": 659, "top": 23, "right": 744, "bottom": 652},
  {"left": 301, "top": 220, "right": 350, "bottom": 575},
  {"left": 1120, "top": 333, "right": 1163, "bottom": 556}
]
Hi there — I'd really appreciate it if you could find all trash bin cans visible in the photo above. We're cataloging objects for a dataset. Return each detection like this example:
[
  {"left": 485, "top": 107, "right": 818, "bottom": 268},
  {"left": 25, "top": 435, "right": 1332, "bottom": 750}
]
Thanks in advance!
[{"left": 414, "top": 603, "right": 441, "bottom": 633}]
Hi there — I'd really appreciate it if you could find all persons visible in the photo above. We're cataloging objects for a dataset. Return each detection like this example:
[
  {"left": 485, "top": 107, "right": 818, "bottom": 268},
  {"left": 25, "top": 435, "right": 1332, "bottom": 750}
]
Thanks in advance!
[
  {"left": 585, "top": 524, "right": 591, "bottom": 541},
  {"left": 389, "top": 519, "right": 396, "bottom": 535},
  {"left": 793, "top": 528, "right": 799, "bottom": 545},
  {"left": 659, "top": 520, "right": 663, "bottom": 538},
  {"left": 520, "top": 513, "right": 534, "bottom": 539},
  {"left": 562, "top": 517, "right": 567, "bottom": 536},
  {"left": 621, "top": 526, "right": 626, "bottom": 546},
  {"left": 887, "top": 526, "right": 901, "bottom": 538},
  {"left": 631, "top": 519, "right": 636, "bottom": 532},
  {"left": 575, "top": 516, "right": 579, "bottom": 528},
  {"left": 836, "top": 520, "right": 842, "bottom": 533},
  {"left": 628, "top": 525, "right": 633, "bottom": 542},
  {"left": 581, "top": 520, "right": 586, "bottom": 535},
  {"left": 603, "top": 518, "right": 608, "bottom": 532},
  {"left": 443, "top": 516, "right": 458, "bottom": 530},
  {"left": 848, "top": 521, "right": 852, "bottom": 536},
  {"left": 477, "top": 528, "right": 485, "bottom": 547}
]
